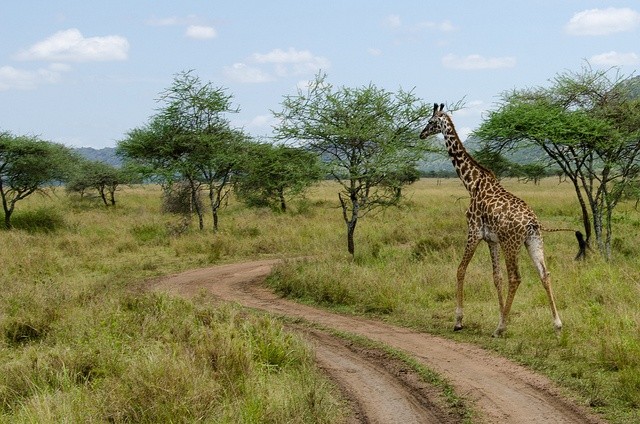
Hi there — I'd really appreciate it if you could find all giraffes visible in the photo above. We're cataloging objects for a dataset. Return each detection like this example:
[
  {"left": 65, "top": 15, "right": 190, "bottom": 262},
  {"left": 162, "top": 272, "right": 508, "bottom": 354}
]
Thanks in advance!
[{"left": 421, "top": 103, "right": 596, "bottom": 349}]
